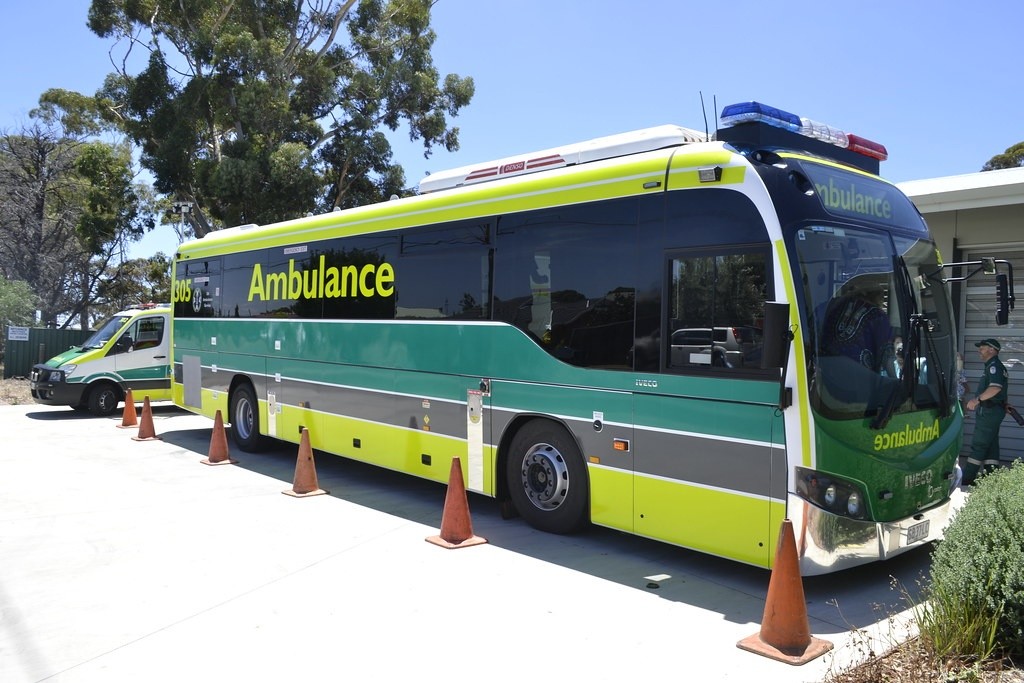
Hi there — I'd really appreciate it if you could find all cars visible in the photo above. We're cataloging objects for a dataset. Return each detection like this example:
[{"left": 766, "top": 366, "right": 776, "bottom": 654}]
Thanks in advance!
[{"left": 527, "top": 302, "right": 650, "bottom": 357}]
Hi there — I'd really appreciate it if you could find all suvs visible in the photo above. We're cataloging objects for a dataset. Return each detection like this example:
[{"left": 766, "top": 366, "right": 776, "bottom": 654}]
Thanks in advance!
[{"left": 631, "top": 326, "right": 761, "bottom": 367}]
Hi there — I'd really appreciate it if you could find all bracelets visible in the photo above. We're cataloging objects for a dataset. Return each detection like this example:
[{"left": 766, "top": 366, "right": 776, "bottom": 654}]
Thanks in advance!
[{"left": 976, "top": 396, "right": 982, "bottom": 402}]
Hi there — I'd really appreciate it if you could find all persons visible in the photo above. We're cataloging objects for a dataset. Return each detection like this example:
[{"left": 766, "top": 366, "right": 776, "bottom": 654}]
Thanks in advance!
[
  {"left": 516, "top": 244, "right": 548, "bottom": 323},
  {"left": 807, "top": 269, "right": 898, "bottom": 378},
  {"left": 879, "top": 335, "right": 969, "bottom": 408},
  {"left": 961, "top": 338, "right": 1013, "bottom": 486}
]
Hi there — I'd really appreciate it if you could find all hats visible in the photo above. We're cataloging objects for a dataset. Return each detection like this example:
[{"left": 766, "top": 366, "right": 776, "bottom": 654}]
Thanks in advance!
[{"left": 974, "top": 338, "right": 1001, "bottom": 351}]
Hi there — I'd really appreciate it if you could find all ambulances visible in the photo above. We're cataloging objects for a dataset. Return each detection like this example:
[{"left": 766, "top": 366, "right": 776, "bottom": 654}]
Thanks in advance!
[
  {"left": 30, "top": 302, "right": 171, "bottom": 416},
  {"left": 170, "top": 103, "right": 1016, "bottom": 578}
]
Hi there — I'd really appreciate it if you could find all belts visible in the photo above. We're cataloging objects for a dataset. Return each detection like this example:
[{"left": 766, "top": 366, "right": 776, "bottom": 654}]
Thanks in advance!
[{"left": 979, "top": 399, "right": 1006, "bottom": 407}]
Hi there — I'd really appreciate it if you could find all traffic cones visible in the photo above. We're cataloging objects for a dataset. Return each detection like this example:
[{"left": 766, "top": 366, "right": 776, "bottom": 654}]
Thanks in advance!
[
  {"left": 116, "top": 387, "right": 140, "bottom": 429},
  {"left": 130, "top": 395, "right": 164, "bottom": 441},
  {"left": 424, "top": 455, "right": 489, "bottom": 550},
  {"left": 280, "top": 428, "right": 331, "bottom": 498},
  {"left": 736, "top": 518, "right": 835, "bottom": 666},
  {"left": 200, "top": 409, "right": 239, "bottom": 466}
]
